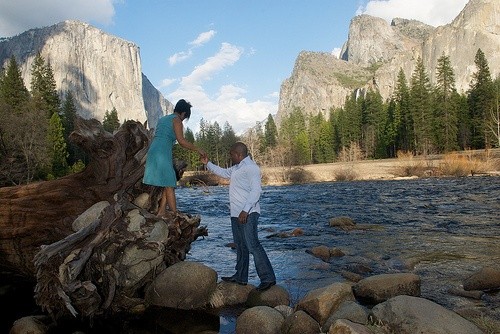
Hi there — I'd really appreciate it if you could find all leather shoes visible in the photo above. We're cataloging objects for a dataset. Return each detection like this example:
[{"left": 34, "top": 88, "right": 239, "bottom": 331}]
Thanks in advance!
[
  {"left": 221, "top": 276, "right": 248, "bottom": 285},
  {"left": 257, "top": 281, "right": 276, "bottom": 292}
]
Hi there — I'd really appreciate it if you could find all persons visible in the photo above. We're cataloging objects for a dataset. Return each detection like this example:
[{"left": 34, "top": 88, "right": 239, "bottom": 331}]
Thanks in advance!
[
  {"left": 141, "top": 99, "right": 208, "bottom": 219},
  {"left": 198, "top": 142, "right": 276, "bottom": 292}
]
